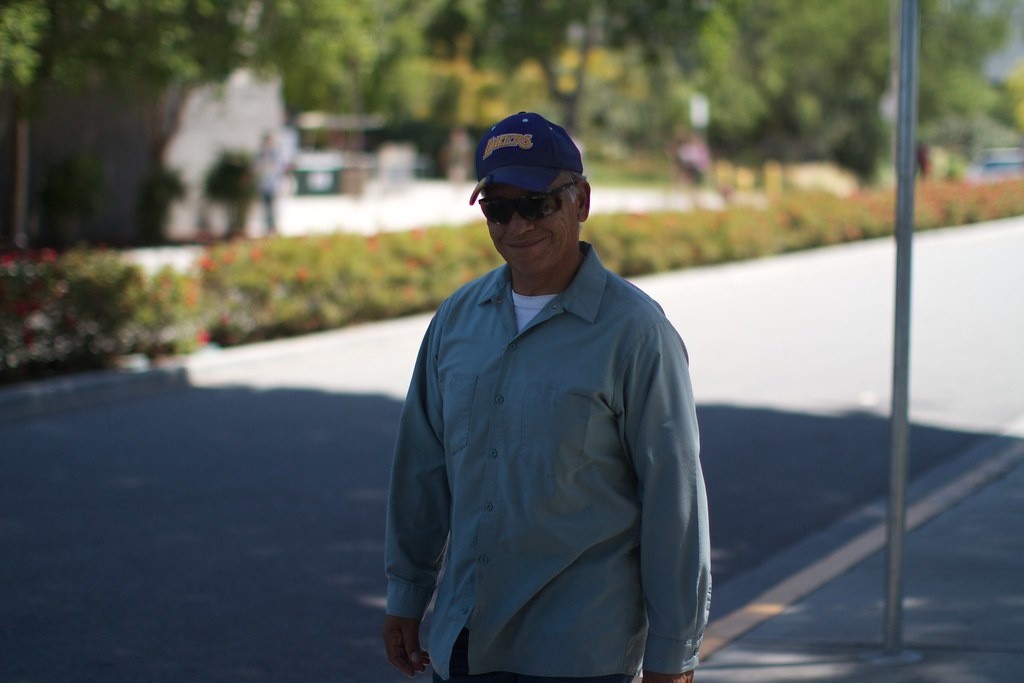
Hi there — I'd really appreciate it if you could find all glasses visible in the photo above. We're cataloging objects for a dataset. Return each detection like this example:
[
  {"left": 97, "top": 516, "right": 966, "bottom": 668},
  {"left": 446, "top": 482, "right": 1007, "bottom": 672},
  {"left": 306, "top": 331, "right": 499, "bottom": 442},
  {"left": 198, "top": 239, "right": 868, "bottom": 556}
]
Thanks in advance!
[{"left": 478, "top": 179, "right": 582, "bottom": 224}]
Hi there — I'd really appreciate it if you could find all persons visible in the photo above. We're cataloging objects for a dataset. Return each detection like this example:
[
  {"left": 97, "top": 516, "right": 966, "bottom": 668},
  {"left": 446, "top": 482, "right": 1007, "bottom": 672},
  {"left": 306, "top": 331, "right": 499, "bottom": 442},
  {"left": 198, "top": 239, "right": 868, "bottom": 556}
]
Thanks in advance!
[
  {"left": 383, "top": 110, "right": 710, "bottom": 683},
  {"left": 257, "top": 134, "right": 292, "bottom": 232},
  {"left": 668, "top": 123, "right": 709, "bottom": 209}
]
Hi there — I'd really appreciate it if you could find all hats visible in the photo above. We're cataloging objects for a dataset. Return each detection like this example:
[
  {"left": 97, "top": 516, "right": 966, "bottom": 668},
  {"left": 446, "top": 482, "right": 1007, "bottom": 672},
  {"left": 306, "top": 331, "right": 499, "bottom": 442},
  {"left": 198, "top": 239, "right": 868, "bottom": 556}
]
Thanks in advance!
[{"left": 469, "top": 111, "right": 583, "bottom": 205}]
